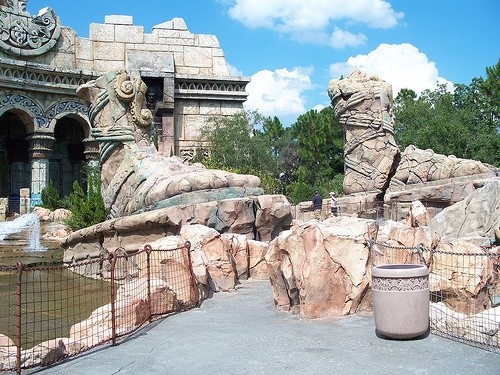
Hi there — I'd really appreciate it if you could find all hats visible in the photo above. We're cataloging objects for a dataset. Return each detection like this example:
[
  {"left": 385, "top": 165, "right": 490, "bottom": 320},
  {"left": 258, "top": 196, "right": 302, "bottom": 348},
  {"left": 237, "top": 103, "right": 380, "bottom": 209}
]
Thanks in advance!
[{"left": 328, "top": 191, "right": 335, "bottom": 195}]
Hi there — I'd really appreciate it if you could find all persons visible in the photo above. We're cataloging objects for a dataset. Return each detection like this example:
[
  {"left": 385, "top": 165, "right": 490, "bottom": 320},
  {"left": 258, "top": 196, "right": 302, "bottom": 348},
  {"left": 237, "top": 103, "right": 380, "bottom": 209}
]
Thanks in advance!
[
  {"left": 312, "top": 190, "right": 323, "bottom": 220},
  {"left": 328, "top": 191, "right": 338, "bottom": 217}
]
